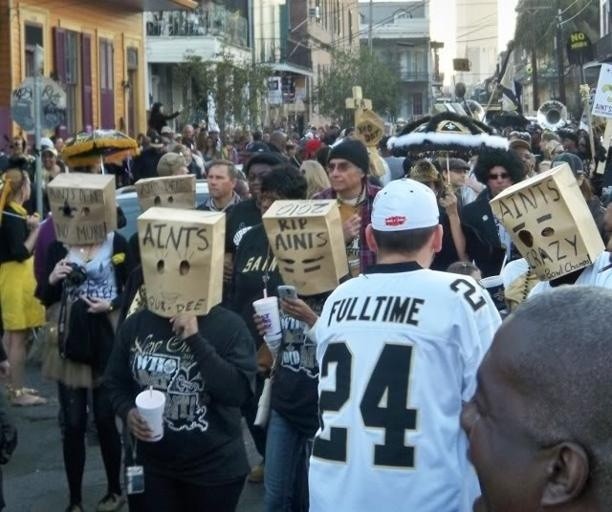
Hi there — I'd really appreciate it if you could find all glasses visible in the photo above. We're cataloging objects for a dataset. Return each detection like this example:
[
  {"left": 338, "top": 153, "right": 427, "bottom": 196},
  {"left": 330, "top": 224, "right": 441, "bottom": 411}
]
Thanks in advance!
[
  {"left": 488, "top": 173, "right": 511, "bottom": 180},
  {"left": 326, "top": 162, "right": 358, "bottom": 172}
]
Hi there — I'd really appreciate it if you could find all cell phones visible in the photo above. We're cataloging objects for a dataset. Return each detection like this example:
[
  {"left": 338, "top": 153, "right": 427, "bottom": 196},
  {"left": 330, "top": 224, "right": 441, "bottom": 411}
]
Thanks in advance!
[{"left": 278, "top": 285, "right": 297, "bottom": 301}]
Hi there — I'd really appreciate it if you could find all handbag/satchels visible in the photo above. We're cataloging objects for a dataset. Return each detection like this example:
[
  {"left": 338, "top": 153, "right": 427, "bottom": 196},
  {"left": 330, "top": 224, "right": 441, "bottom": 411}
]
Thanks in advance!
[{"left": 252, "top": 376, "right": 272, "bottom": 431}]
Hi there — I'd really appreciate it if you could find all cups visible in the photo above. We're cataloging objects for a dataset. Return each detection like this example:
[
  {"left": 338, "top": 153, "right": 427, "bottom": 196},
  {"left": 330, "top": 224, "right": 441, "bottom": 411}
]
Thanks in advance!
[
  {"left": 481, "top": 276, "right": 506, "bottom": 313},
  {"left": 253, "top": 297, "right": 281, "bottom": 343},
  {"left": 135, "top": 389, "right": 165, "bottom": 442}
]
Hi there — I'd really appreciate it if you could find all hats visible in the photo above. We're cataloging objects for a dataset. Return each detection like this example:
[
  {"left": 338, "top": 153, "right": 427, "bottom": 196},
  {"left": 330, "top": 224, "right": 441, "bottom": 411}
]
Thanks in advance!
[
  {"left": 148, "top": 121, "right": 224, "bottom": 150},
  {"left": 371, "top": 178, "right": 439, "bottom": 233},
  {"left": 328, "top": 138, "right": 369, "bottom": 174},
  {"left": 43, "top": 148, "right": 58, "bottom": 156},
  {"left": 551, "top": 152, "right": 584, "bottom": 178},
  {"left": 509, "top": 139, "right": 530, "bottom": 151},
  {"left": 41, "top": 137, "right": 54, "bottom": 148},
  {"left": 441, "top": 158, "right": 471, "bottom": 171}
]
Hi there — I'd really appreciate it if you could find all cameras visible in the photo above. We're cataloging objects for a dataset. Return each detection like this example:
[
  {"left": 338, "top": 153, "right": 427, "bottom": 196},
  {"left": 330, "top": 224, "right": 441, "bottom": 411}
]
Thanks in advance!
[
  {"left": 126, "top": 465, "right": 145, "bottom": 494},
  {"left": 65, "top": 263, "right": 87, "bottom": 287}
]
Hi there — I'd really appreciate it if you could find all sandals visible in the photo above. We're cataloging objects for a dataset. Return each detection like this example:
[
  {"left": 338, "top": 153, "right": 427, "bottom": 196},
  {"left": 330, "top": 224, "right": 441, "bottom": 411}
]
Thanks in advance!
[{"left": 5, "top": 383, "right": 48, "bottom": 407}]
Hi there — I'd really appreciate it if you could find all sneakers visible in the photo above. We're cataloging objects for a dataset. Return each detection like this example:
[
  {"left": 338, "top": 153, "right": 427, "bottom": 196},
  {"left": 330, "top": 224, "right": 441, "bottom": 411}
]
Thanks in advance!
[
  {"left": 96, "top": 491, "right": 126, "bottom": 512},
  {"left": 66, "top": 504, "right": 83, "bottom": 512}
]
